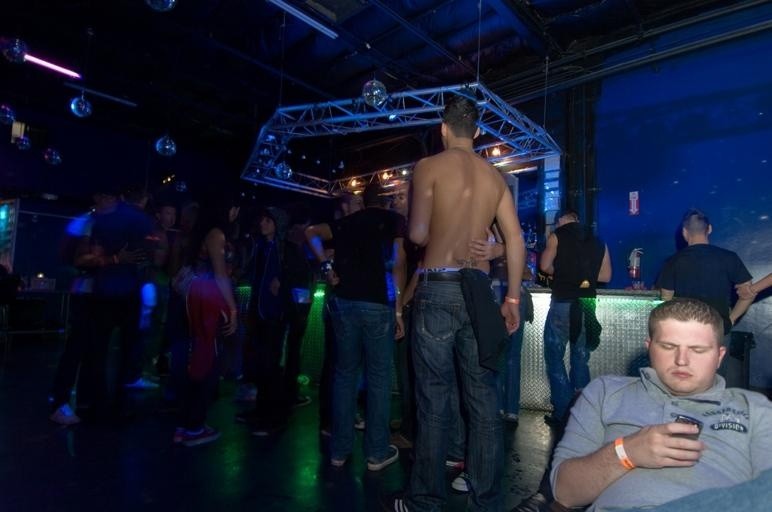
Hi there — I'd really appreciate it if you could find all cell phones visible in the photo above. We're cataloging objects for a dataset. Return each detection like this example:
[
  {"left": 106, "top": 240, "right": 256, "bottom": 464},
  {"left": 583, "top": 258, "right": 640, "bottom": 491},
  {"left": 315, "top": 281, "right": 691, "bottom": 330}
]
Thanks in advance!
[{"left": 673, "top": 415, "right": 703, "bottom": 440}]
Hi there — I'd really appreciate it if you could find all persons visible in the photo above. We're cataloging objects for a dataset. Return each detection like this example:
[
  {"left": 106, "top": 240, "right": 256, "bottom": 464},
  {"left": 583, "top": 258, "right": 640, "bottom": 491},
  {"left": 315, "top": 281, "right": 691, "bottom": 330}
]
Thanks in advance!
[
  {"left": 549, "top": 297, "right": 769, "bottom": 512},
  {"left": 655, "top": 208, "right": 757, "bottom": 388},
  {"left": 736, "top": 273, "right": 772, "bottom": 300}
]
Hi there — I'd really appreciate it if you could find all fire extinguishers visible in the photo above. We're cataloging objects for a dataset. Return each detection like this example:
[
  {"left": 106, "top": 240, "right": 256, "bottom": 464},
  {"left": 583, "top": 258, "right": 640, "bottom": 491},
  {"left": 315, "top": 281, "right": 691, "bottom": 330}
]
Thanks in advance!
[{"left": 627, "top": 248, "right": 643, "bottom": 279}]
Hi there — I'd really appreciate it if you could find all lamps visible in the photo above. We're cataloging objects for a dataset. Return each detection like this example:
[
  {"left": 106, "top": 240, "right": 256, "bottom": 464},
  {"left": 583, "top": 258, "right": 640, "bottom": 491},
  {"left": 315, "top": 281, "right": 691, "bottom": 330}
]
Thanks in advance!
[
  {"left": 4, "top": 38, "right": 24, "bottom": 64},
  {"left": 26, "top": 54, "right": 80, "bottom": 77},
  {"left": 70, "top": 90, "right": 91, "bottom": 117},
  {"left": 3, "top": 107, "right": 67, "bottom": 169},
  {"left": 261, "top": 133, "right": 294, "bottom": 179},
  {"left": 271, "top": 1, "right": 338, "bottom": 39},
  {"left": 148, "top": 1, "right": 175, "bottom": 12},
  {"left": 363, "top": 72, "right": 386, "bottom": 106},
  {"left": 349, "top": 167, "right": 414, "bottom": 195},
  {"left": 162, "top": 174, "right": 188, "bottom": 195},
  {"left": 484, "top": 146, "right": 512, "bottom": 169},
  {"left": 156, "top": 128, "right": 177, "bottom": 156}
]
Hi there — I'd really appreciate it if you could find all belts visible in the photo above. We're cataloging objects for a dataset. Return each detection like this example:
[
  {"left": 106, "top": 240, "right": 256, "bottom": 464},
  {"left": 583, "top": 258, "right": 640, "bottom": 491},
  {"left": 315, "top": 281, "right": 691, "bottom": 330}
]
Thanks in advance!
[{"left": 419, "top": 272, "right": 460, "bottom": 282}]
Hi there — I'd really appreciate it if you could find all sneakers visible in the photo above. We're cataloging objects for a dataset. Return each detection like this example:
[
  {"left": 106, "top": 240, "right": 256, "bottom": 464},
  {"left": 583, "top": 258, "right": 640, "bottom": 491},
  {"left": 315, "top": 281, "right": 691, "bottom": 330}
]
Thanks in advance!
[
  {"left": 381, "top": 494, "right": 410, "bottom": 512},
  {"left": 171, "top": 412, "right": 287, "bottom": 446},
  {"left": 321, "top": 412, "right": 414, "bottom": 471},
  {"left": 452, "top": 474, "right": 473, "bottom": 492},
  {"left": 445, "top": 460, "right": 464, "bottom": 469},
  {"left": 503, "top": 413, "right": 519, "bottom": 423},
  {"left": 545, "top": 414, "right": 565, "bottom": 427},
  {"left": 291, "top": 394, "right": 313, "bottom": 410}
]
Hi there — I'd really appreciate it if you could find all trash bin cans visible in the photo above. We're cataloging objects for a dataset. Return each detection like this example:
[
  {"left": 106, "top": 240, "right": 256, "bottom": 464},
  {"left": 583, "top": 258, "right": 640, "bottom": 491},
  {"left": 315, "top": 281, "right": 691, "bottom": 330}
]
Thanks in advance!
[{"left": 725, "top": 331, "right": 755, "bottom": 389}]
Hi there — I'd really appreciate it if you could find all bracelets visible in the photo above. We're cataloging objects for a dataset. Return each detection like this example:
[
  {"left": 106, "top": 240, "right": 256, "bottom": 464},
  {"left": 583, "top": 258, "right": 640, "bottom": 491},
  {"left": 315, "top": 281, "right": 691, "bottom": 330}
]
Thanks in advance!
[
  {"left": 614, "top": 436, "right": 635, "bottom": 471},
  {"left": 747, "top": 284, "right": 756, "bottom": 297}
]
